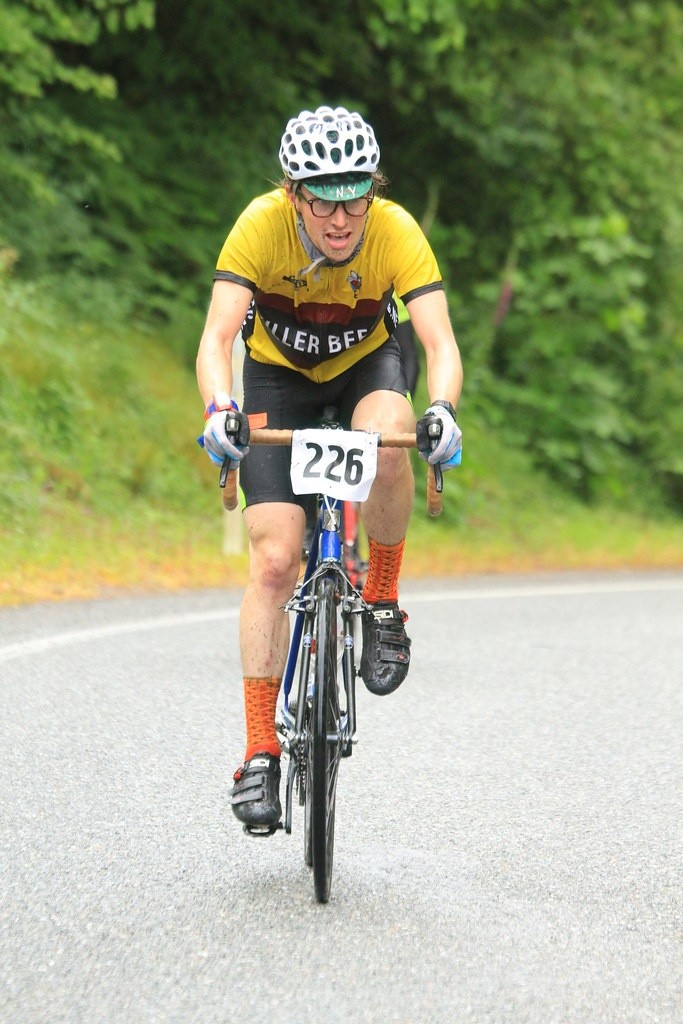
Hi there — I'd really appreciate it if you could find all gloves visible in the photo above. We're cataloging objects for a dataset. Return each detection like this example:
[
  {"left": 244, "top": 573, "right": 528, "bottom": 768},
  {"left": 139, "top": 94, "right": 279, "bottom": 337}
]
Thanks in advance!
[
  {"left": 197, "top": 396, "right": 250, "bottom": 470},
  {"left": 415, "top": 399, "right": 464, "bottom": 472}
]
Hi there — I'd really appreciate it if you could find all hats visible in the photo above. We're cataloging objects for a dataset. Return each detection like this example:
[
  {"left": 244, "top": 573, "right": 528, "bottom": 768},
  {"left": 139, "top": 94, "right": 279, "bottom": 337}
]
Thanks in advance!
[{"left": 301, "top": 171, "right": 375, "bottom": 200}]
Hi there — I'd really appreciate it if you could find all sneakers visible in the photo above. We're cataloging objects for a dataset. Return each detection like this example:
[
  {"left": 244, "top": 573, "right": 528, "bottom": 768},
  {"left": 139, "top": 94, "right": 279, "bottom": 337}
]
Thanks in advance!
[
  {"left": 361, "top": 600, "right": 412, "bottom": 695},
  {"left": 228, "top": 750, "right": 283, "bottom": 826}
]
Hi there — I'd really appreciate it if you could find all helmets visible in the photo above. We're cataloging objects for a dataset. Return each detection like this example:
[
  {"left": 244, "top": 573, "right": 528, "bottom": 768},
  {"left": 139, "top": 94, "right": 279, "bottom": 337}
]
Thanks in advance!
[{"left": 279, "top": 106, "right": 381, "bottom": 181}]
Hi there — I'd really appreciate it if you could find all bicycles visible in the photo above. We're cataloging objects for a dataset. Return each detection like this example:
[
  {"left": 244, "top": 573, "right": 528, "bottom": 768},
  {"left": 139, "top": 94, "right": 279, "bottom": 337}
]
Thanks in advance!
[{"left": 219, "top": 412, "right": 442, "bottom": 903}]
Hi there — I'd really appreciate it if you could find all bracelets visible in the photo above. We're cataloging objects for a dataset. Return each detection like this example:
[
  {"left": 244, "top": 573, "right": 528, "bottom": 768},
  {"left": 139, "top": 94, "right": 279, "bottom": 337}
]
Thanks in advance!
[
  {"left": 204, "top": 400, "right": 238, "bottom": 420},
  {"left": 431, "top": 400, "right": 457, "bottom": 422}
]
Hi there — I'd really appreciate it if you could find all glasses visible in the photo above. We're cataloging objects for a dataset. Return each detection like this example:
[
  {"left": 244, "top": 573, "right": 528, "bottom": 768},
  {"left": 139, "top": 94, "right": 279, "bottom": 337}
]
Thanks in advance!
[{"left": 296, "top": 183, "right": 375, "bottom": 217}]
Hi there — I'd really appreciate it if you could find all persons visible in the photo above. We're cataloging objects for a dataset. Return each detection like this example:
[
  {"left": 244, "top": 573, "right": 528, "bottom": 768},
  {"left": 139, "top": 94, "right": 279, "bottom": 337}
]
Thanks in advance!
[{"left": 195, "top": 106, "right": 464, "bottom": 829}]
